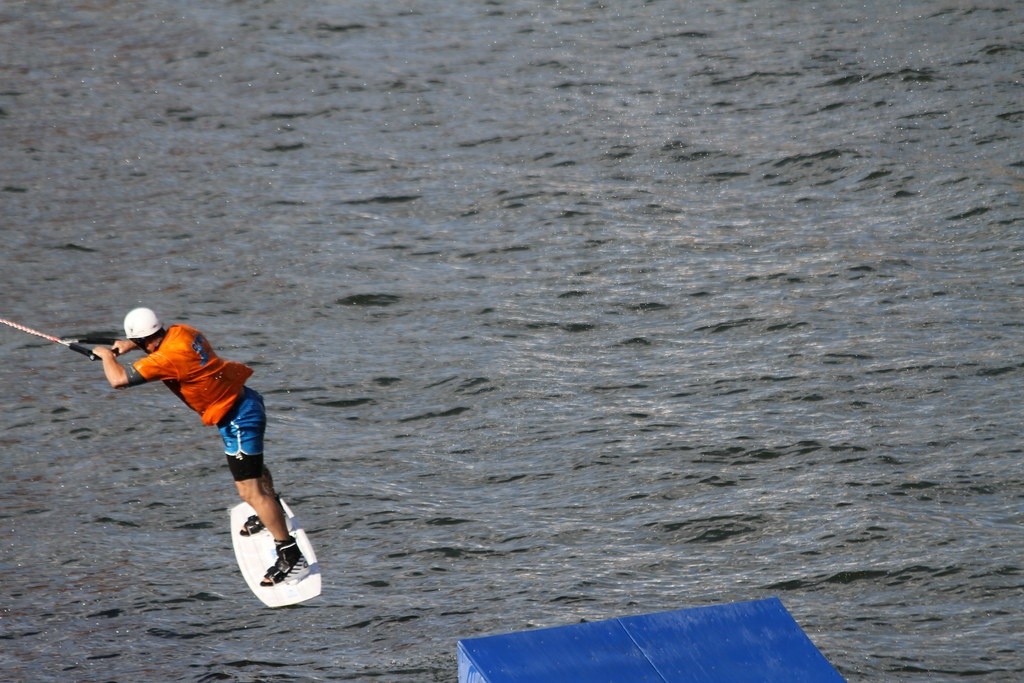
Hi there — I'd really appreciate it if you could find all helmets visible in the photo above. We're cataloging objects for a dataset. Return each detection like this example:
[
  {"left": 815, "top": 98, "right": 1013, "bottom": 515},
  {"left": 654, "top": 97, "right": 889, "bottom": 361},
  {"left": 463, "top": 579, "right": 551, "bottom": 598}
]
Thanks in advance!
[{"left": 123, "top": 307, "right": 163, "bottom": 340}]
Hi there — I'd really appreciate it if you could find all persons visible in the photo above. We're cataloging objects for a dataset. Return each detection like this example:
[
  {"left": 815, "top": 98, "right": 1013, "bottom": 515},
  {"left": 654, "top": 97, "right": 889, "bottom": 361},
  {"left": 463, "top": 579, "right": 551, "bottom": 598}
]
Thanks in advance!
[{"left": 93, "top": 307, "right": 310, "bottom": 588}]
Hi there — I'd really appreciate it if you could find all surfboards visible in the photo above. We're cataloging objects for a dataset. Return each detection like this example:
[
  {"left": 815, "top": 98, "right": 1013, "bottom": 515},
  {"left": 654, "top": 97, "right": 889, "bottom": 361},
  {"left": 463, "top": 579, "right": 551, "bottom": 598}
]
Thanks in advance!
[{"left": 227, "top": 493, "right": 324, "bottom": 610}]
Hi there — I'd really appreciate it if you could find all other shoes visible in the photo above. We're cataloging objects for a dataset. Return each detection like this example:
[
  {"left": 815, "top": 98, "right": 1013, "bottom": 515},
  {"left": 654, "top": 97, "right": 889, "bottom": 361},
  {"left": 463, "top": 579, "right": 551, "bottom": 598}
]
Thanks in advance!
[
  {"left": 239, "top": 492, "right": 285, "bottom": 536},
  {"left": 260, "top": 536, "right": 302, "bottom": 587}
]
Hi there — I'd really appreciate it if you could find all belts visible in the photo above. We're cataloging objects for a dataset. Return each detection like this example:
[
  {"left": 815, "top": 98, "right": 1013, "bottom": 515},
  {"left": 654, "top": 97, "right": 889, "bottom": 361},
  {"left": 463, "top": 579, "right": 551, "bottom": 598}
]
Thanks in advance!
[{"left": 216, "top": 385, "right": 246, "bottom": 429}]
